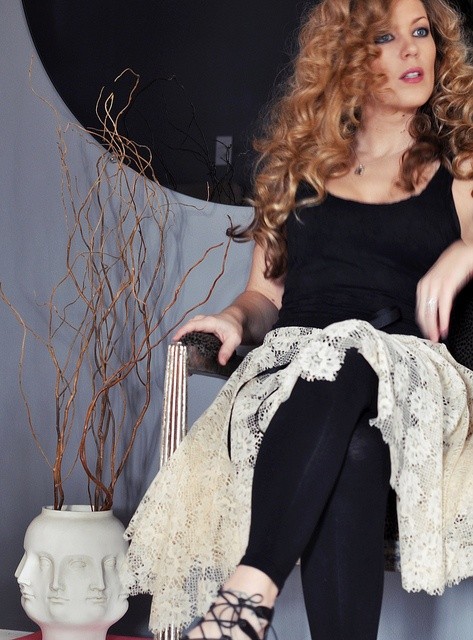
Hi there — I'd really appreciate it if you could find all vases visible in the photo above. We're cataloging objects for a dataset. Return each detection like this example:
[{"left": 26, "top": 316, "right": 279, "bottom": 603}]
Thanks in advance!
[{"left": 13, "top": 505, "right": 129, "bottom": 640}]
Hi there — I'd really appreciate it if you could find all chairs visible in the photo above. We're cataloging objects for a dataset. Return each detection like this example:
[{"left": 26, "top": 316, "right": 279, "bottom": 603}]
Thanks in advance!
[{"left": 156, "top": 344, "right": 399, "bottom": 576}]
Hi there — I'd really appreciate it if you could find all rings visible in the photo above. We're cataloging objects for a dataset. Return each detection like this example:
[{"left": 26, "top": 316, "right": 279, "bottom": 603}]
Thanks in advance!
[{"left": 425, "top": 299, "right": 436, "bottom": 304}]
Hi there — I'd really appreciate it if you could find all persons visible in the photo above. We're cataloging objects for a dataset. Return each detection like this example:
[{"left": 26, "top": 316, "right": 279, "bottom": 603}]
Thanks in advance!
[{"left": 165, "top": 0, "right": 472, "bottom": 638}]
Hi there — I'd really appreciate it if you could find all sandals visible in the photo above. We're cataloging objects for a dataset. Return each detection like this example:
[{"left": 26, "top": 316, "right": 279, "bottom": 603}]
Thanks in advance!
[{"left": 177, "top": 588, "right": 278, "bottom": 640}]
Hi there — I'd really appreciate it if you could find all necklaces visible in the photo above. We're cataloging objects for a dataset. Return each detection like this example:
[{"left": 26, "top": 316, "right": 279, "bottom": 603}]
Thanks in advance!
[{"left": 352, "top": 148, "right": 408, "bottom": 176}]
